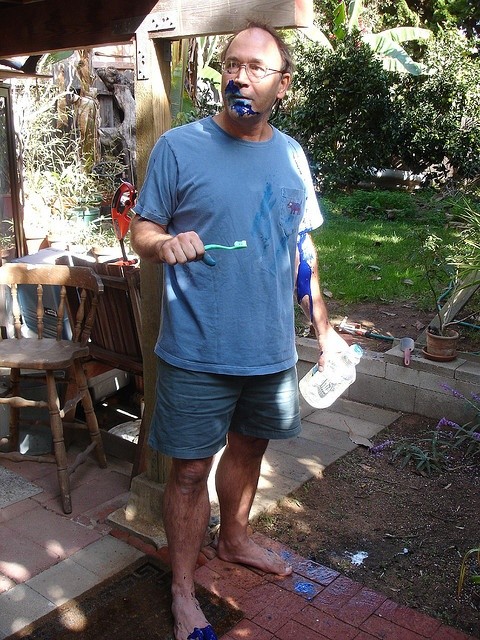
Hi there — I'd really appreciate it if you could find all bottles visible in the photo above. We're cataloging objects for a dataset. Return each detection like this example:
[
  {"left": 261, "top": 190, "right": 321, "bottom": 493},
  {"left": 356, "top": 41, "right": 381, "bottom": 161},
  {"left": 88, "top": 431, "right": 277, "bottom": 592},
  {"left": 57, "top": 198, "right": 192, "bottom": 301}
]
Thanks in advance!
[{"left": 299, "top": 344, "right": 363, "bottom": 409}]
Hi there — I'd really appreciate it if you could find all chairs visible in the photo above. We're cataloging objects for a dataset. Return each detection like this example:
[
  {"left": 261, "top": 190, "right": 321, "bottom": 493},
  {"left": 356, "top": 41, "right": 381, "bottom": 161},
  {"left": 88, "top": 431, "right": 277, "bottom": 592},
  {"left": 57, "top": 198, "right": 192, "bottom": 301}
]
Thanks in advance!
[
  {"left": 0, "top": 263, "right": 108, "bottom": 513},
  {"left": 63, "top": 253, "right": 150, "bottom": 491}
]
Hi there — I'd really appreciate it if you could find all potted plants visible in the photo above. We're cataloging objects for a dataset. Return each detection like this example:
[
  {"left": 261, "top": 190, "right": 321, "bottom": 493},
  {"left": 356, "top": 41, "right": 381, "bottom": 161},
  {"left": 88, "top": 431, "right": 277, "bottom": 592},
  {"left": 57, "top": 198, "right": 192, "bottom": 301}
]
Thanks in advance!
[{"left": 420, "top": 229, "right": 460, "bottom": 362}]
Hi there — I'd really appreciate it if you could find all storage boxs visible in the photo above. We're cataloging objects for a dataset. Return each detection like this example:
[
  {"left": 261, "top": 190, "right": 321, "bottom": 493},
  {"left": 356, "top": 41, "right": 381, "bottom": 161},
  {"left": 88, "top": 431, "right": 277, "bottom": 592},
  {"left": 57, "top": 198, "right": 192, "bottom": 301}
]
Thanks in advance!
[{"left": 87, "top": 367, "right": 129, "bottom": 400}]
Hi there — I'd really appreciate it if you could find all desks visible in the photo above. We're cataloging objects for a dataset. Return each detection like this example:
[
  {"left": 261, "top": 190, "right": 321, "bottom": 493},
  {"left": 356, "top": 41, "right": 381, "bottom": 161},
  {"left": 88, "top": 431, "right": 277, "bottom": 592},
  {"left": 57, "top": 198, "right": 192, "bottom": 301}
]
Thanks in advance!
[{"left": 1, "top": 247, "right": 139, "bottom": 385}]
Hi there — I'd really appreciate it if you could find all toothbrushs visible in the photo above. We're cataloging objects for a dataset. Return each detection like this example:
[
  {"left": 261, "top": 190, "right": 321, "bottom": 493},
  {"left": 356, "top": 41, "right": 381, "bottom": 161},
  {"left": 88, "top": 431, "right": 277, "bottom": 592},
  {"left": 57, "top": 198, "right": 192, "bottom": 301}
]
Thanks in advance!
[{"left": 204, "top": 241, "right": 248, "bottom": 251}]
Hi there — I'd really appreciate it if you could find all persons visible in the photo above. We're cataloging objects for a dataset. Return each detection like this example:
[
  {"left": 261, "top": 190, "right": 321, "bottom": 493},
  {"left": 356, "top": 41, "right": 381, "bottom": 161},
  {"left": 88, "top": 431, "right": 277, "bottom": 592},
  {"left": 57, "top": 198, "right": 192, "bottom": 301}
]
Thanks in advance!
[{"left": 130, "top": 16, "right": 357, "bottom": 639}]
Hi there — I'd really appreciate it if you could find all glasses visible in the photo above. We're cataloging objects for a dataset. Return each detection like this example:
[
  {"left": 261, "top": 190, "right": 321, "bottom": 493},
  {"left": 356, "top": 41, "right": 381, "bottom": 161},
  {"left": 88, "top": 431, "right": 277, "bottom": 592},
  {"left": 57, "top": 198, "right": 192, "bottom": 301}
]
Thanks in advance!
[{"left": 221, "top": 60, "right": 282, "bottom": 79}]
